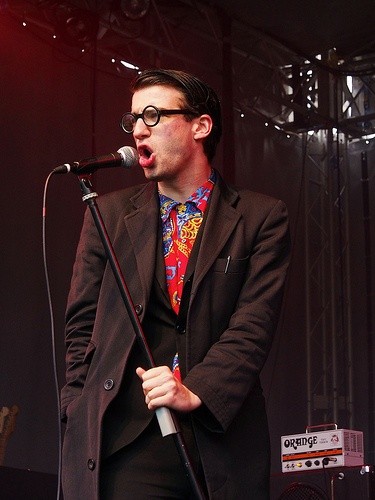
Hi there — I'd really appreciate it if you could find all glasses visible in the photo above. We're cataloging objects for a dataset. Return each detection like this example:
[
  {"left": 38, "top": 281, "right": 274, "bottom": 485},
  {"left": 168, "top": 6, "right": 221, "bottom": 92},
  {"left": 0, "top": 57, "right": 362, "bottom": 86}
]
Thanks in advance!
[{"left": 119, "top": 105, "right": 199, "bottom": 134}]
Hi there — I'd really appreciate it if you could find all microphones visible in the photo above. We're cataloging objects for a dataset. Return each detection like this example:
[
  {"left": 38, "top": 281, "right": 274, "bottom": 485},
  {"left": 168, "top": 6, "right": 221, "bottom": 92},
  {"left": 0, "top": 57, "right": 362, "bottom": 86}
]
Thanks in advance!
[{"left": 53, "top": 145, "right": 139, "bottom": 174}]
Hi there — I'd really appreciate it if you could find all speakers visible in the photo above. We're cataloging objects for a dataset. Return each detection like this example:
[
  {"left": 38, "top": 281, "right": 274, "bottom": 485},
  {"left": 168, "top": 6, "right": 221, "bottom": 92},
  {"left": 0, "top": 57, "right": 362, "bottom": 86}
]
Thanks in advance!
[{"left": 269, "top": 465, "right": 370, "bottom": 500}]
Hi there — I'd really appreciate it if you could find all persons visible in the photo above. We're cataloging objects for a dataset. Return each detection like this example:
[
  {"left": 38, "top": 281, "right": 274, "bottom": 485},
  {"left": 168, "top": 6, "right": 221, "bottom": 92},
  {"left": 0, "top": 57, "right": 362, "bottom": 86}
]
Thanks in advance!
[{"left": 60, "top": 68, "right": 291, "bottom": 500}]
